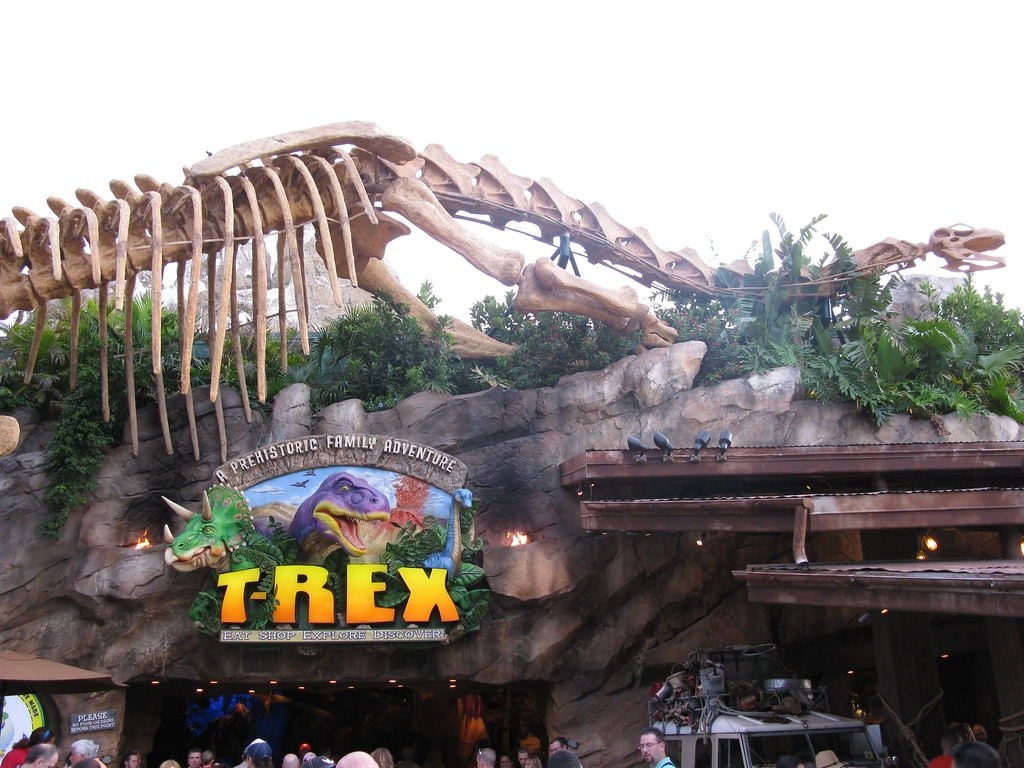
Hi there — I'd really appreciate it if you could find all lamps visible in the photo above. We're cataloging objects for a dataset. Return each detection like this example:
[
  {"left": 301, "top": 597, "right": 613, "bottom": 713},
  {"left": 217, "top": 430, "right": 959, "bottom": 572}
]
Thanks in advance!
[
  {"left": 627, "top": 436, "right": 647, "bottom": 456},
  {"left": 653, "top": 432, "right": 675, "bottom": 457},
  {"left": 719, "top": 429, "right": 733, "bottom": 456},
  {"left": 694, "top": 431, "right": 711, "bottom": 456}
]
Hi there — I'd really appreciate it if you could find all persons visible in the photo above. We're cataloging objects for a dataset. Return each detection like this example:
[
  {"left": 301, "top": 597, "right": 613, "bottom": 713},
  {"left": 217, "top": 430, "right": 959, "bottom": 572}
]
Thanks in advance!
[{"left": 0, "top": 726, "right": 1024, "bottom": 768}]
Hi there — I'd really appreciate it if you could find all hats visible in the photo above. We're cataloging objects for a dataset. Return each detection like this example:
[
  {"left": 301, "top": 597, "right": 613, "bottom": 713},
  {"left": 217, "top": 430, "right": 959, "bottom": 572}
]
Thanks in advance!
[
  {"left": 304, "top": 756, "right": 336, "bottom": 768},
  {"left": 247, "top": 741, "right": 272, "bottom": 761},
  {"left": 299, "top": 743, "right": 310, "bottom": 751},
  {"left": 815, "top": 750, "right": 845, "bottom": 768}
]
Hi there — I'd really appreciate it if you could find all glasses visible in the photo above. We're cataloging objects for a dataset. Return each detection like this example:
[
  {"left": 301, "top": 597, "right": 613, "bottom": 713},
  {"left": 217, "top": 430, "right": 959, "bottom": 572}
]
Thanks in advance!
[
  {"left": 478, "top": 746, "right": 483, "bottom": 755},
  {"left": 638, "top": 743, "right": 659, "bottom": 750},
  {"left": 551, "top": 747, "right": 560, "bottom": 752}
]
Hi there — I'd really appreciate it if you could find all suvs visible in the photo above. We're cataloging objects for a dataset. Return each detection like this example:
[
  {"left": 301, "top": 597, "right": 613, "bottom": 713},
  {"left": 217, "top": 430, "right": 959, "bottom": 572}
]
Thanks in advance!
[{"left": 651, "top": 709, "right": 887, "bottom": 768}]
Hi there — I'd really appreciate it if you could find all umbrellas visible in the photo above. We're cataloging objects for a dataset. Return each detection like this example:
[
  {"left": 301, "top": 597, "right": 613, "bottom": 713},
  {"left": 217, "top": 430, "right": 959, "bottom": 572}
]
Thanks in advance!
[{"left": 0, "top": 649, "right": 129, "bottom": 725}]
